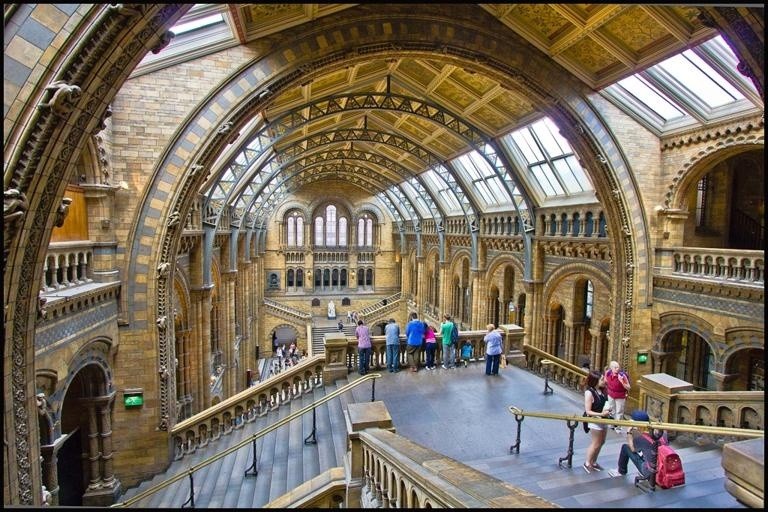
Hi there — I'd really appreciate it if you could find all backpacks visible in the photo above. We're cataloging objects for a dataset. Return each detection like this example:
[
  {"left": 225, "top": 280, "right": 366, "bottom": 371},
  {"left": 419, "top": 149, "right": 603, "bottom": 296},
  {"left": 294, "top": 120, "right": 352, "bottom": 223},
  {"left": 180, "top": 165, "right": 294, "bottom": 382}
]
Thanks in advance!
[
  {"left": 641, "top": 431, "right": 685, "bottom": 488},
  {"left": 450, "top": 322, "right": 458, "bottom": 343},
  {"left": 606, "top": 369, "right": 630, "bottom": 384}
]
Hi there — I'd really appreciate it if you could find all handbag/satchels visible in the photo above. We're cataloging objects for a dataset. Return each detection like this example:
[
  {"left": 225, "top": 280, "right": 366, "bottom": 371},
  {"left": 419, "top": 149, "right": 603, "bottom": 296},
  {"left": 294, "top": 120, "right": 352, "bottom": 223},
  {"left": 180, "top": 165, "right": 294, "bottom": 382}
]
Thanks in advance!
[
  {"left": 582, "top": 412, "right": 590, "bottom": 434},
  {"left": 498, "top": 353, "right": 506, "bottom": 369}
]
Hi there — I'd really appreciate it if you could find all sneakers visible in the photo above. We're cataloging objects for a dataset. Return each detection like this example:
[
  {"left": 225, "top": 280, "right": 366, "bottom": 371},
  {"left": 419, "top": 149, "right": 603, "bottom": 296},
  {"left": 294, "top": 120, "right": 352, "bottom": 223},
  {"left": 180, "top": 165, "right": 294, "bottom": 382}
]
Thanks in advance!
[
  {"left": 388, "top": 369, "right": 401, "bottom": 373},
  {"left": 358, "top": 370, "right": 369, "bottom": 375},
  {"left": 583, "top": 462, "right": 605, "bottom": 474},
  {"left": 449, "top": 364, "right": 456, "bottom": 368},
  {"left": 441, "top": 365, "right": 448, "bottom": 369},
  {"left": 425, "top": 366, "right": 435, "bottom": 371},
  {"left": 608, "top": 469, "right": 623, "bottom": 477},
  {"left": 410, "top": 368, "right": 417, "bottom": 372}
]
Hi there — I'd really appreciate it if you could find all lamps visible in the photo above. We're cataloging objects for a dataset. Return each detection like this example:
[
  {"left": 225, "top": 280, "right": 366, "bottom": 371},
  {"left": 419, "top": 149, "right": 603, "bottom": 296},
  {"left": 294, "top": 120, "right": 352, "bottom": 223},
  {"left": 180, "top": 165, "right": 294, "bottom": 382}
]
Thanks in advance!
[{"left": 508, "top": 302, "right": 516, "bottom": 313}]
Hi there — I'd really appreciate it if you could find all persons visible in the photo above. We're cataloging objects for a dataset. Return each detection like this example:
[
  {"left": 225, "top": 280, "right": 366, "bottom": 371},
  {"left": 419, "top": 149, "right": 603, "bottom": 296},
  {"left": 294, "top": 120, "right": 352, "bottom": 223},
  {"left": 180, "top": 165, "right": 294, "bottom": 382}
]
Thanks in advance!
[
  {"left": 583, "top": 361, "right": 669, "bottom": 482},
  {"left": 269, "top": 336, "right": 296, "bottom": 377},
  {"left": 382, "top": 297, "right": 387, "bottom": 306},
  {"left": 347, "top": 310, "right": 356, "bottom": 325},
  {"left": 337, "top": 320, "right": 344, "bottom": 333},
  {"left": 355, "top": 312, "right": 503, "bottom": 376}
]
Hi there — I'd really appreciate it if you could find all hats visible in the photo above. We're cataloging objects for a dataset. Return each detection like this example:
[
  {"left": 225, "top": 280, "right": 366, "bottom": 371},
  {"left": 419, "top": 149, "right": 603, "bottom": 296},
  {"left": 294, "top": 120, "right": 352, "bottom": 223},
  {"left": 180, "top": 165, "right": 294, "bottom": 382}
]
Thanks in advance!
[{"left": 631, "top": 411, "right": 649, "bottom": 421}]
